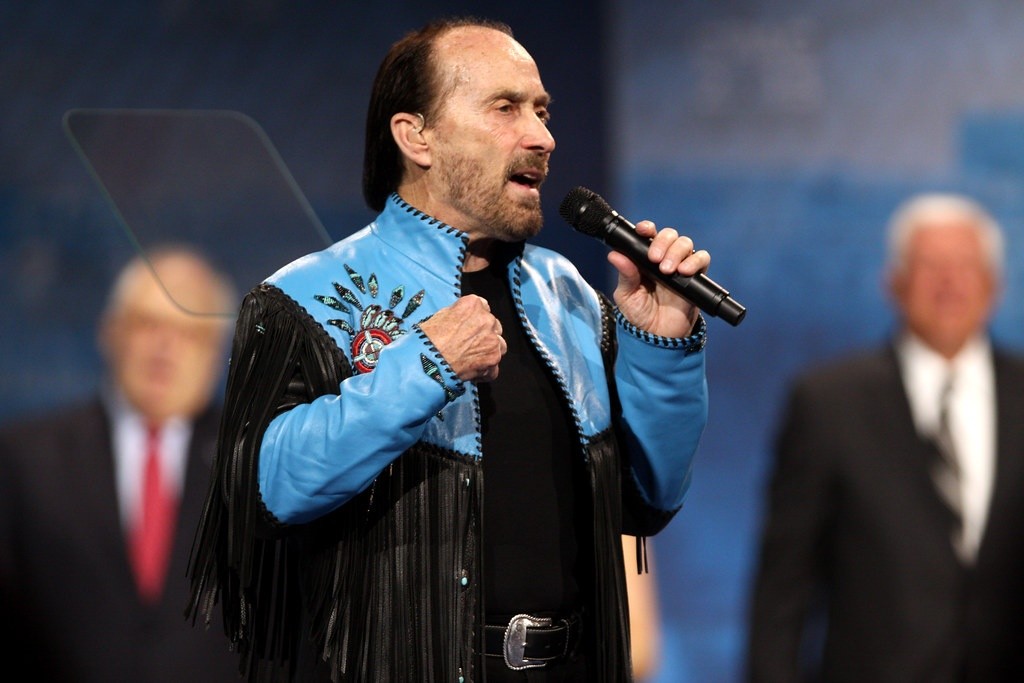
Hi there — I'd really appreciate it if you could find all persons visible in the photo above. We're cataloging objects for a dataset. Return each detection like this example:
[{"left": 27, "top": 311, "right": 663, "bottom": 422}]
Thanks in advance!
[
  {"left": 0, "top": 253, "right": 242, "bottom": 683},
  {"left": 186, "top": 23, "right": 711, "bottom": 683},
  {"left": 749, "top": 197, "right": 1024, "bottom": 683}
]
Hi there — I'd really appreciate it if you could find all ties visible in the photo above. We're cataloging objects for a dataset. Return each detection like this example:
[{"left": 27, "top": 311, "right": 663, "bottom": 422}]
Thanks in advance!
[
  {"left": 930, "top": 362, "right": 966, "bottom": 565},
  {"left": 127, "top": 420, "right": 182, "bottom": 599}
]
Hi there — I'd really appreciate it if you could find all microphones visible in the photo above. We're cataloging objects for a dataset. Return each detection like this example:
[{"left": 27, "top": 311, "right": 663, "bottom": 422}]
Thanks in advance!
[{"left": 559, "top": 185, "right": 747, "bottom": 327}]
